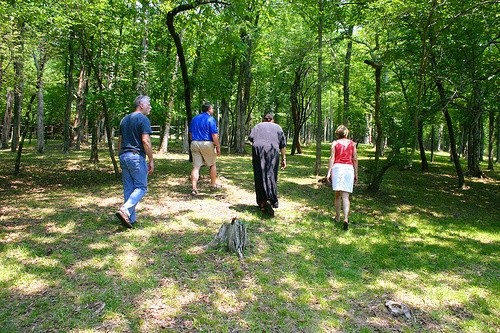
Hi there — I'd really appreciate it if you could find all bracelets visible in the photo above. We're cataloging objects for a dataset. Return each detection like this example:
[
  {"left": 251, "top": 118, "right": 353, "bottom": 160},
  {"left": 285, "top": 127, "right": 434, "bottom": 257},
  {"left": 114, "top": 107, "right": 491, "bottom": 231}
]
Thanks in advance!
[
  {"left": 282, "top": 158, "right": 286, "bottom": 160},
  {"left": 355, "top": 174, "right": 358, "bottom": 177}
]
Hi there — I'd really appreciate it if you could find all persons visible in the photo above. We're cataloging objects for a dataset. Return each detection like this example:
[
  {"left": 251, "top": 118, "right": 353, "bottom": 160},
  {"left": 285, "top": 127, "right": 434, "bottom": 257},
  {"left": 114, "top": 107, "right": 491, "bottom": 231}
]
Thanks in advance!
[
  {"left": 326, "top": 125, "right": 359, "bottom": 231},
  {"left": 249, "top": 114, "right": 287, "bottom": 216},
  {"left": 116, "top": 95, "right": 155, "bottom": 230},
  {"left": 188, "top": 102, "right": 223, "bottom": 196}
]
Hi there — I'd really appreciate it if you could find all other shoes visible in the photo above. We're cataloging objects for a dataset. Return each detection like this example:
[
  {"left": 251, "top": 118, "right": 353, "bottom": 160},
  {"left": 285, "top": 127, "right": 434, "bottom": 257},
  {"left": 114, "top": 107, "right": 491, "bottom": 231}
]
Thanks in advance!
[
  {"left": 273, "top": 201, "right": 278, "bottom": 208},
  {"left": 191, "top": 189, "right": 198, "bottom": 195},
  {"left": 116, "top": 211, "right": 133, "bottom": 228},
  {"left": 343, "top": 221, "right": 348, "bottom": 230},
  {"left": 210, "top": 184, "right": 224, "bottom": 191},
  {"left": 261, "top": 206, "right": 265, "bottom": 212},
  {"left": 334, "top": 216, "right": 340, "bottom": 222}
]
